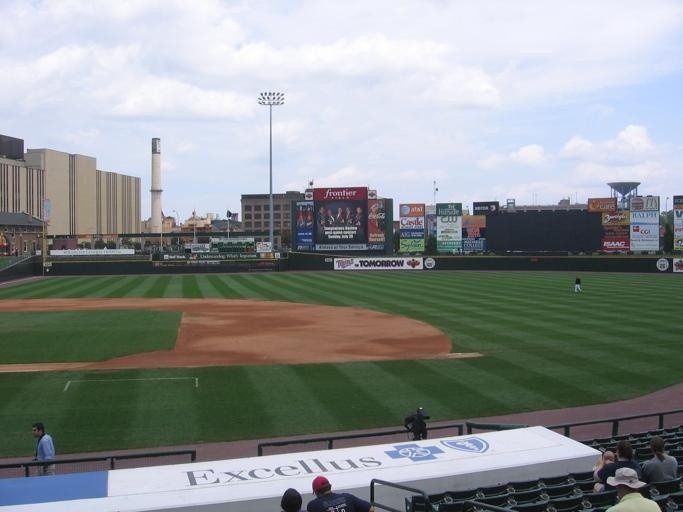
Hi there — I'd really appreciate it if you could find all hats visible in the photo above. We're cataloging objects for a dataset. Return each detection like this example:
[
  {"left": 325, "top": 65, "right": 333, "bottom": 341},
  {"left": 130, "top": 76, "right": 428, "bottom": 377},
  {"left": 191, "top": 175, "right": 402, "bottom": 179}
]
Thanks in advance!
[
  {"left": 606, "top": 466, "right": 647, "bottom": 489},
  {"left": 311, "top": 475, "right": 330, "bottom": 495}
]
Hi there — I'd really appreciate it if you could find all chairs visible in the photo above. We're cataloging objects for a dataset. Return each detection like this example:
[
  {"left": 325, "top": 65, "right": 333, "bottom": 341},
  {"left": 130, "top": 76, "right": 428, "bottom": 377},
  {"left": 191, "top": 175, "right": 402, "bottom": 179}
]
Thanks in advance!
[{"left": 405, "top": 424, "right": 682, "bottom": 512}]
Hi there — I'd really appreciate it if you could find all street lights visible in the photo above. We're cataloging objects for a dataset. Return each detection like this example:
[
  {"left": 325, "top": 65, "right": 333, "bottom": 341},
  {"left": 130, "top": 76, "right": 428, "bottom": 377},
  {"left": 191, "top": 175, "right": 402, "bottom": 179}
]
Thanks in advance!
[
  {"left": 257, "top": 91, "right": 285, "bottom": 253},
  {"left": 665, "top": 197, "right": 669, "bottom": 214}
]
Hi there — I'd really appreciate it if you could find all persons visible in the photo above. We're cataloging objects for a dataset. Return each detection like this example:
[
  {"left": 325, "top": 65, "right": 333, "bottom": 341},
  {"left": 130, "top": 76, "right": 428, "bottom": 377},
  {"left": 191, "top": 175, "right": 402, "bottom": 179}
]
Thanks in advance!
[
  {"left": 307, "top": 476, "right": 373, "bottom": 512},
  {"left": 641, "top": 435, "right": 678, "bottom": 482},
  {"left": 605, "top": 467, "right": 661, "bottom": 512},
  {"left": 575, "top": 277, "right": 582, "bottom": 292},
  {"left": 593, "top": 441, "right": 643, "bottom": 493},
  {"left": 32, "top": 423, "right": 56, "bottom": 476},
  {"left": 604, "top": 450, "right": 614, "bottom": 463},
  {"left": 281, "top": 488, "right": 307, "bottom": 512},
  {"left": 315, "top": 205, "right": 365, "bottom": 228}
]
